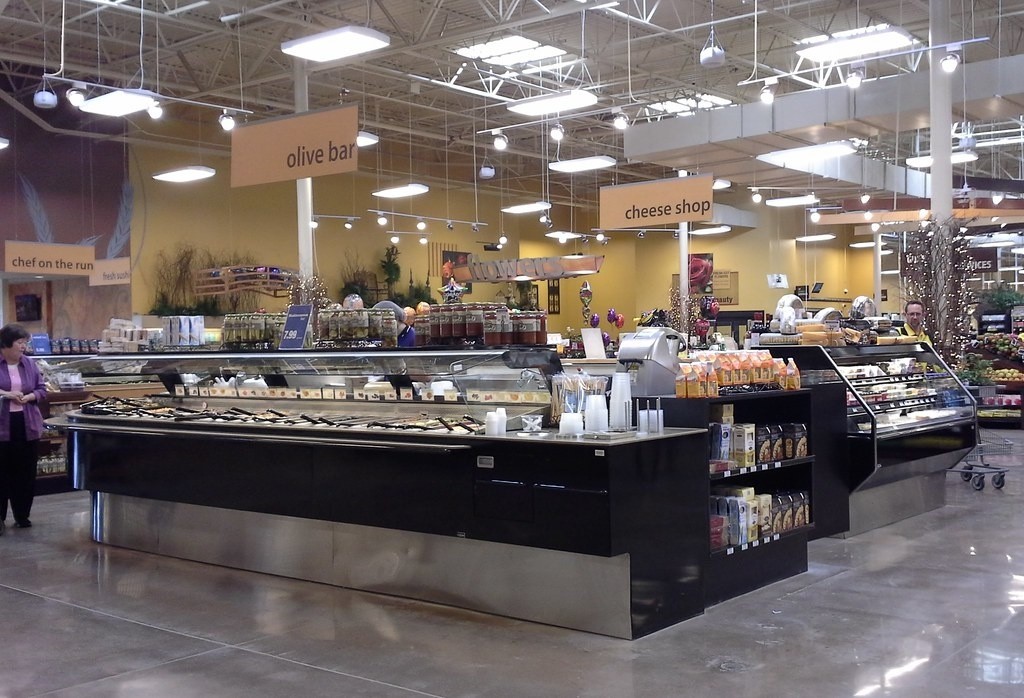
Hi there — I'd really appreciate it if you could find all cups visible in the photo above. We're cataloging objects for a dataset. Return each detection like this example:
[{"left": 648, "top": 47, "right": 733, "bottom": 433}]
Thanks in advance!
[
  {"left": 485, "top": 408, "right": 506, "bottom": 437},
  {"left": 521, "top": 415, "right": 544, "bottom": 431},
  {"left": 559, "top": 412, "right": 583, "bottom": 434},
  {"left": 610, "top": 373, "right": 633, "bottom": 428},
  {"left": 640, "top": 410, "right": 664, "bottom": 432},
  {"left": 584, "top": 395, "right": 609, "bottom": 432}
]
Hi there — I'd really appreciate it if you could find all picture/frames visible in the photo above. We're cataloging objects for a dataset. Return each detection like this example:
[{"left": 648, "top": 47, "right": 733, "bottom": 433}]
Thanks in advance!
[
  {"left": 442, "top": 250, "right": 472, "bottom": 294},
  {"left": 881, "top": 290, "right": 888, "bottom": 301}
]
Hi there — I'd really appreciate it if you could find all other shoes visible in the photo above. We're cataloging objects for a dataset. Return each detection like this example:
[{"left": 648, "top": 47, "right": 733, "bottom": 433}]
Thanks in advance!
[
  {"left": 14, "top": 519, "right": 31, "bottom": 528},
  {"left": 0, "top": 521, "right": 5, "bottom": 536}
]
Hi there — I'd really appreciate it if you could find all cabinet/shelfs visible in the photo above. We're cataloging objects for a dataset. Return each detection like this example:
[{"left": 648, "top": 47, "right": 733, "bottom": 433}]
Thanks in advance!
[
  {"left": 967, "top": 348, "right": 1024, "bottom": 430},
  {"left": 750, "top": 340, "right": 979, "bottom": 540},
  {"left": 29, "top": 353, "right": 166, "bottom": 497},
  {"left": 976, "top": 309, "right": 1011, "bottom": 334},
  {"left": 637, "top": 393, "right": 816, "bottom": 608},
  {"left": 41, "top": 353, "right": 809, "bottom": 647},
  {"left": 1011, "top": 306, "right": 1024, "bottom": 334}
]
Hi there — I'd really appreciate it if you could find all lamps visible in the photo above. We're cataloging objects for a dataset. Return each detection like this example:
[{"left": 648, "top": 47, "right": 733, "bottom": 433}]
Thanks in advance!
[
  {"left": 539, "top": 212, "right": 553, "bottom": 229},
  {"left": 217, "top": 109, "right": 237, "bottom": 131},
  {"left": 65, "top": 87, "right": 164, "bottom": 121},
  {"left": 454, "top": 33, "right": 569, "bottom": 67},
  {"left": 151, "top": 164, "right": 216, "bottom": 183},
  {"left": 501, "top": 200, "right": 552, "bottom": 215},
  {"left": 357, "top": 131, "right": 379, "bottom": 148},
  {"left": 390, "top": 233, "right": 400, "bottom": 244},
  {"left": 508, "top": 87, "right": 599, "bottom": 140},
  {"left": 496, "top": 233, "right": 508, "bottom": 250},
  {"left": 377, "top": 213, "right": 388, "bottom": 226},
  {"left": 33, "top": 1, "right": 59, "bottom": 109},
  {"left": 344, "top": 218, "right": 355, "bottom": 230},
  {"left": 544, "top": 231, "right": 606, "bottom": 245},
  {"left": 418, "top": 234, "right": 428, "bottom": 245},
  {"left": 549, "top": 154, "right": 617, "bottom": 174},
  {"left": 371, "top": 182, "right": 430, "bottom": 201},
  {"left": 491, "top": 130, "right": 510, "bottom": 150},
  {"left": 613, "top": 0, "right": 1024, "bottom": 252},
  {"left": 0, "top": 137, "right": 9, "bottom": 149},
  {"left": 311, "top": 221, "right": 319, "bottom": 228},
  {"left": 415, "top": 218, "right": 426, "bottom": 230},
  {"left": 279, "top": 23, "right": 390, "bottom": 63}
]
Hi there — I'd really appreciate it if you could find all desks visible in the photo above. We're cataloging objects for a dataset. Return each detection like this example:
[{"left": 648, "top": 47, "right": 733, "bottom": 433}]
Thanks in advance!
[{"left": 965, "top": 384, "right": 1006, "bottom": 466}]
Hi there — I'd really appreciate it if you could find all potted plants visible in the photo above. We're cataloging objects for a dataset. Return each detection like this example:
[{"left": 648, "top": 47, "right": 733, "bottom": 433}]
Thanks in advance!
[{"left": 955, "top": 371, "right": 970, "bottom": 385}]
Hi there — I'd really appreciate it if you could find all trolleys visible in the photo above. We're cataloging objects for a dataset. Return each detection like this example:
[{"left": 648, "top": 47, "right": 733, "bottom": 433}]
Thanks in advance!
[{"left": 960, "top": 425, "right": 1014, "bottom": 491}]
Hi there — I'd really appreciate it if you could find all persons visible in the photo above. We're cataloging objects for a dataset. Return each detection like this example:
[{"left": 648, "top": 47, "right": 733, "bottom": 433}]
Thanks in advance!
[
  {"left": 0, "top": 323, "right": 47, "bottom": 535},
  {"left": 372, "top": 301, "right": 416, "bottom": 348},
  {"left": 890, "top": 301, "right": 934, "bottom": 348}
]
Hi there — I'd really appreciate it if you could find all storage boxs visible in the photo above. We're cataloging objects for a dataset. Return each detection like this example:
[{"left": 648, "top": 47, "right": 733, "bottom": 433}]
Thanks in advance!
[
  {"left": 170, "top": 316, "right": 177, "bottom": 345},
  {"left": 669, "top": 346, "right": 801, "bottom": 398},
  {"left": 190, "top": 316, "right": 200, "bottom": 345},
  {"left": 161, "top": 315, "right": 167, "bottom": 345},
  {"left": 707, "top": 403, "right": 809, "bottom": 473},
  {"left": 200, "top": 315, "right": 206, "bottom": 345},
  {"left": 977, "top": 395, "right": 1021, "bottom": 418},
  {"left": 710, "top": 483, "right": 810, "bottom": 550},
  {"left": 177, "top": 316, "right": 190, "bottom": 345},
  {"left": 165, "top": 317, "right": 173, "bottom": 345}
]
